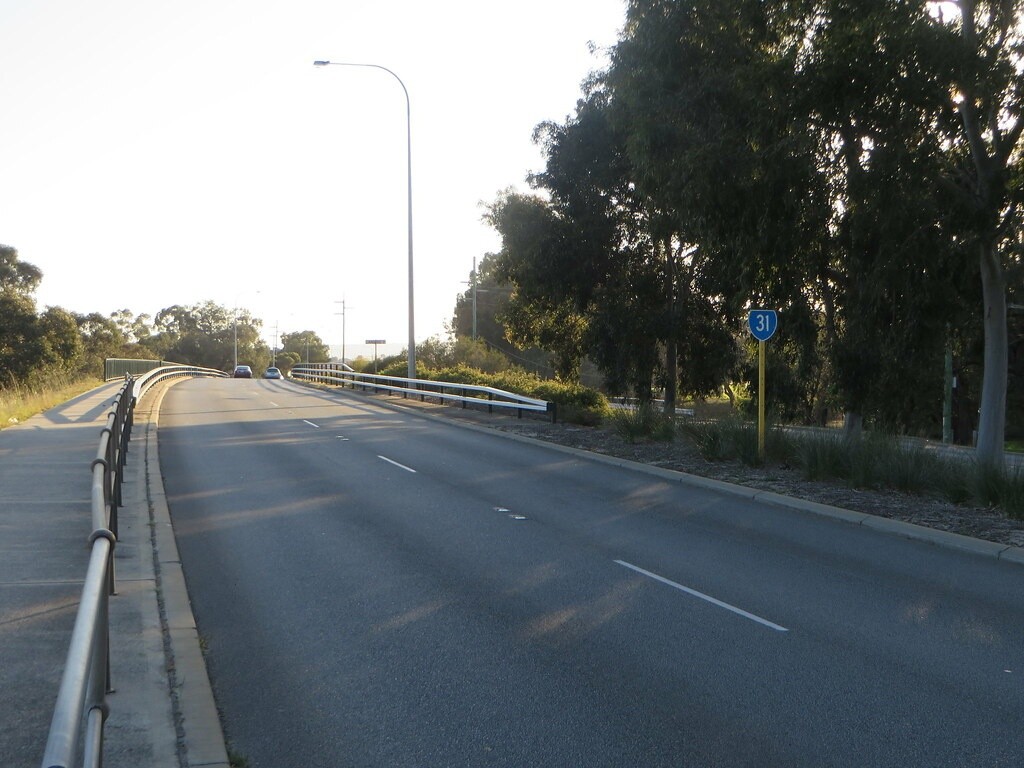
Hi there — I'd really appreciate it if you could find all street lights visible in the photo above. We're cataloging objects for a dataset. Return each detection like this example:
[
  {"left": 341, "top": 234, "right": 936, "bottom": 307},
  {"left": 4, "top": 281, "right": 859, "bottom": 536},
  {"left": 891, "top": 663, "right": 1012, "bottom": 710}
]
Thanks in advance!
[{"left": 312, "top": 59, "right": 418, "bottom": 401}]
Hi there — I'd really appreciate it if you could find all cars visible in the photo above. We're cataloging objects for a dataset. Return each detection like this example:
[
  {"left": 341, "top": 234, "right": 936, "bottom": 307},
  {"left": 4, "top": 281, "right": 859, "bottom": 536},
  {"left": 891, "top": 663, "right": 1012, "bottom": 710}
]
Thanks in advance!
[
  {"left": 233, "top": 365, "right": 252, "bottom": 379},
  {"left": 264, "top": 367, "right": 281, "bottom": 379}
]
